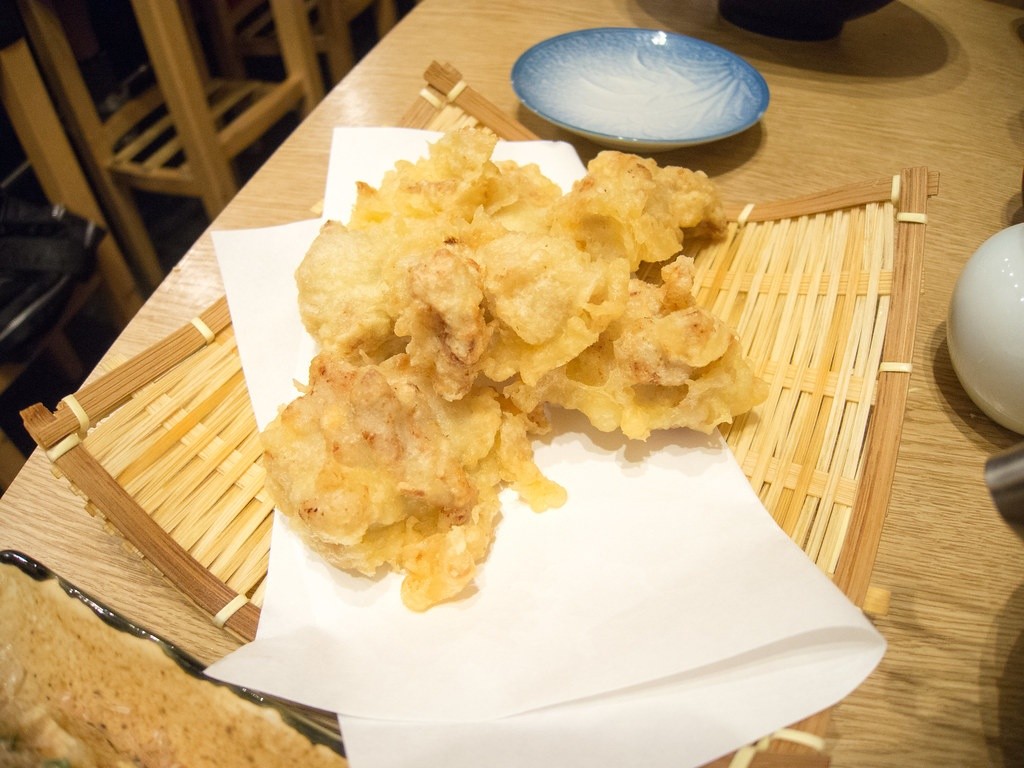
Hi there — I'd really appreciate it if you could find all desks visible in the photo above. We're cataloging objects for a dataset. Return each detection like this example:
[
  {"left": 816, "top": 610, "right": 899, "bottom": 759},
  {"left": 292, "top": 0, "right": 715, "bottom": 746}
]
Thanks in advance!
[{"left": 0, "top": 0, "right": 1024, "bottom": 768}]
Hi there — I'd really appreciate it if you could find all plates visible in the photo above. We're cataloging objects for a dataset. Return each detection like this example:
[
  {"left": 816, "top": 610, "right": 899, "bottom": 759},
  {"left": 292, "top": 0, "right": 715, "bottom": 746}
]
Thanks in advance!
[{"left": 510, "top": 27, "right": 770, "bottom": 157}]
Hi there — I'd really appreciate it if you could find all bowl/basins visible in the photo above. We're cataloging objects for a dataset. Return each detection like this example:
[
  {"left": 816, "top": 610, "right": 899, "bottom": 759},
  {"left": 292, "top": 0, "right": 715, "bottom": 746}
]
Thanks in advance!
[{"left": 716, "top": 0, "right": 897, "bottom": 45}]
